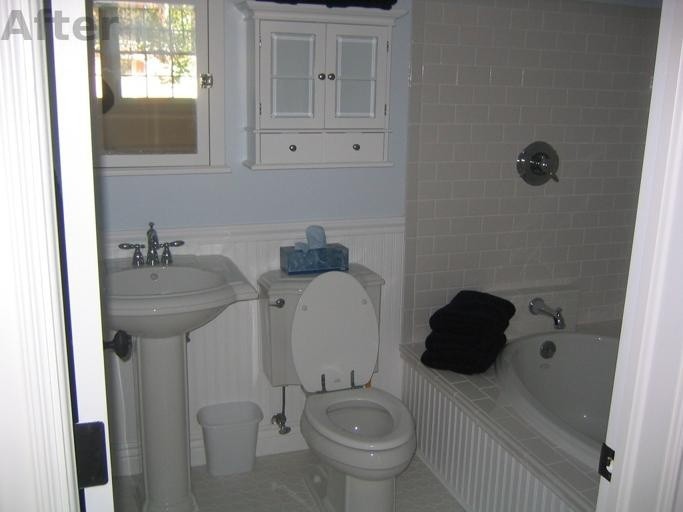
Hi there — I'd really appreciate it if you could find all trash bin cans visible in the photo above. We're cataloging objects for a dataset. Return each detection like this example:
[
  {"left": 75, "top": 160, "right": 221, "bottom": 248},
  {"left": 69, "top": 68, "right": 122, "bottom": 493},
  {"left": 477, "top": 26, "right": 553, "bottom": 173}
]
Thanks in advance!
[{"left": 197, "top": 401, "right": 263, "bottom": 476}]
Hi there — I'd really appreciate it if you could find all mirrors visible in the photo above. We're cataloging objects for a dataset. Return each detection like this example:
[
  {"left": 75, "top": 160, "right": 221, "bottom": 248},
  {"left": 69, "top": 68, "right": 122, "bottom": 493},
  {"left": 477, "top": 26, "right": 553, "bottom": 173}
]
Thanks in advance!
[{"left": 86, "top": 0, "right": 209, "bottom": 167}]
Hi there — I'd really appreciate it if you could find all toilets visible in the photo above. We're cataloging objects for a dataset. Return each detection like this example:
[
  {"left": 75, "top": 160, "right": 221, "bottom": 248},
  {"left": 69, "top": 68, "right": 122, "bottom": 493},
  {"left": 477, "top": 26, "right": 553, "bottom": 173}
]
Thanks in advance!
[{"left": 256, "top": 260, "right": 418, "bottom": 511}]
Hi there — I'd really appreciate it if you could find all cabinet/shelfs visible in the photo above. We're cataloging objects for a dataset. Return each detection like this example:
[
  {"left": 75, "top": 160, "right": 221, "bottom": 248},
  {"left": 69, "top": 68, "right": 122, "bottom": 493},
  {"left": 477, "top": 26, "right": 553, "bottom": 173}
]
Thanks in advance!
[{"left": 235, "top": 0, "right": 410, "bottom": 171}]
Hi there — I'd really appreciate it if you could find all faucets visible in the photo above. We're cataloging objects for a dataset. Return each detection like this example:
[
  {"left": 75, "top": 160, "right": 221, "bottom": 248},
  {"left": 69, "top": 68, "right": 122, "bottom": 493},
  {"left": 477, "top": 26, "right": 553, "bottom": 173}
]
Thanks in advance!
[{"left": 145, "top": 221, "right": 161, "bottom": 266}]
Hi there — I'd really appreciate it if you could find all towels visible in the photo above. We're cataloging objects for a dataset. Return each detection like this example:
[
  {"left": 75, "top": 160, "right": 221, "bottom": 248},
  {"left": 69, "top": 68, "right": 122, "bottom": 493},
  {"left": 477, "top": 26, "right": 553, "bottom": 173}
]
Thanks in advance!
[{"left": 421, "top": 290, "right": 516, "bottom": 375}]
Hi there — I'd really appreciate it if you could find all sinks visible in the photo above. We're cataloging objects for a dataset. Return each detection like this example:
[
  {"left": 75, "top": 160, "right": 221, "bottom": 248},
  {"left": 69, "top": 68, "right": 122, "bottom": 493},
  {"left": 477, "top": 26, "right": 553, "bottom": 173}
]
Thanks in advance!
[{"left": 97, "top": 255, "right": 260, "bottom": 340}]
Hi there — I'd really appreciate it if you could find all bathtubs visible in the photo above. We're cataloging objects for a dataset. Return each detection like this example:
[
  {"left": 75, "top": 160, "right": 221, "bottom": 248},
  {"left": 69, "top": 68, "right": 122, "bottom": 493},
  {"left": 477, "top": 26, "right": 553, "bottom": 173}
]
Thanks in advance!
[{"left": 491, "top": 333, "right": 631, "bottom": 472}]
